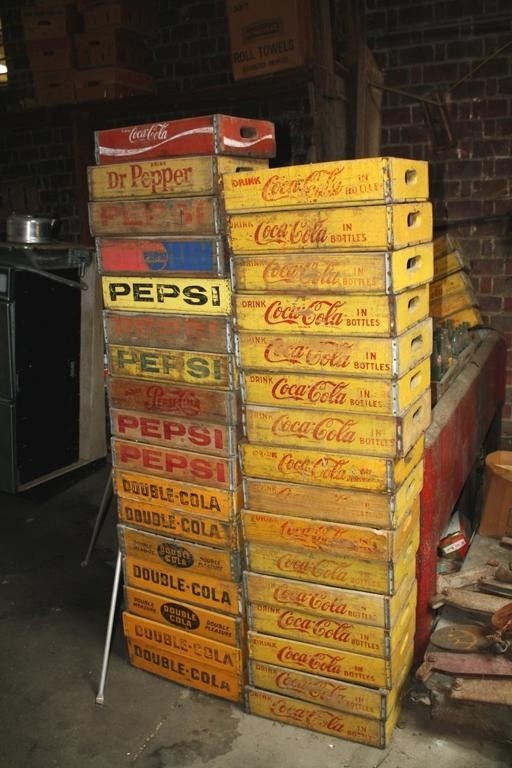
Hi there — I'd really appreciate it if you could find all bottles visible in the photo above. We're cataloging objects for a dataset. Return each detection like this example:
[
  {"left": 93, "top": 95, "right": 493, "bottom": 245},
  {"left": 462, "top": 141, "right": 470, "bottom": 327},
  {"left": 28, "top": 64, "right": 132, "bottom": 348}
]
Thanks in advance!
[{"left": 428, "top": 318, "right": 469, "bottom": 380}]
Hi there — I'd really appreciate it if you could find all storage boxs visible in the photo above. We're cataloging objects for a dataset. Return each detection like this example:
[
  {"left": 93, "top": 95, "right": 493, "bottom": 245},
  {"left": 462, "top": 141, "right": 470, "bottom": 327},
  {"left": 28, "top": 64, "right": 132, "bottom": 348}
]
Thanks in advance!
[{"left": 85, "top": 112, "right": 511, "bottom": 751}]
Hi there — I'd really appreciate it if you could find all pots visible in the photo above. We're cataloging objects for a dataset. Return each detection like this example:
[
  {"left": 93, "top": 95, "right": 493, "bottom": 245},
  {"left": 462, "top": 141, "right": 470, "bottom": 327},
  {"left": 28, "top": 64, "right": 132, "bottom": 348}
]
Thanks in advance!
[{"left": 6, "top": 211, "right": 57, "bottom": 245}]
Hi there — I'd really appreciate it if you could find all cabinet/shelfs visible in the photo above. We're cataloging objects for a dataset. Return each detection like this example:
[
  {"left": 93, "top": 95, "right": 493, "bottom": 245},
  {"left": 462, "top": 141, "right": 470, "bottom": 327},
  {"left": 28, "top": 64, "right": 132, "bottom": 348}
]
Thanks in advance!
[{"left": 2, "top": 241, "right": 111, "bottom": 527}]
